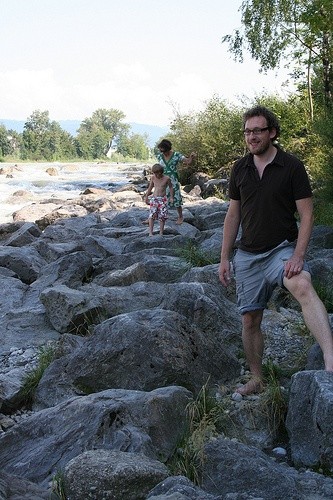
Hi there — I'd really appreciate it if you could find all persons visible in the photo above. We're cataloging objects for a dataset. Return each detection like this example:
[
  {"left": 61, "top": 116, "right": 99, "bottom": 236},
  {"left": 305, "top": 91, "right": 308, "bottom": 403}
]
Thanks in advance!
[
  {"left": 155, "top": 139, "right": 196, "bottom": 226},
  {"left": 218, "top": 105, "right": 333, "bottom": 397},
  {"left": 143, "top": 163, "right": 176, "bottom": 237}
]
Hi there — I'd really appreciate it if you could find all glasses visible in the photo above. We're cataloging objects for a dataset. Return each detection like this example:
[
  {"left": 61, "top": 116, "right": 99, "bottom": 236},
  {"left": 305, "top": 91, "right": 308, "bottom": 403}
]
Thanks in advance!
[{"left": 243, "top": 126, "right": 269, "bottom": 135}]
